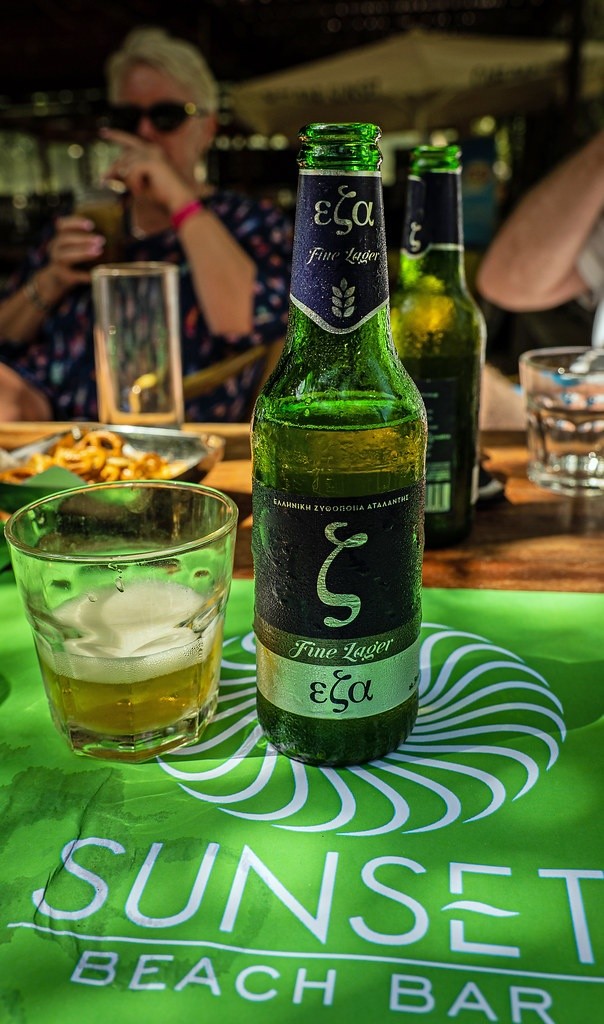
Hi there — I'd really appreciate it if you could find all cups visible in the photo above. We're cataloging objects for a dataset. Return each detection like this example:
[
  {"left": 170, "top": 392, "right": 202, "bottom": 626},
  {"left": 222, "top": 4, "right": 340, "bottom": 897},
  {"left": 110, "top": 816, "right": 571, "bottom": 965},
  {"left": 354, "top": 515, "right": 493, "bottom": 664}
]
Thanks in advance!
[
  {"left": 517, "top": 344, "right": 603, "bottom": 500},
  {"left": 92, "top": 261, "right": 186, "bottom": 433},
  {"left": 4, "top": 479, "right": 239, "bottom": 765}
]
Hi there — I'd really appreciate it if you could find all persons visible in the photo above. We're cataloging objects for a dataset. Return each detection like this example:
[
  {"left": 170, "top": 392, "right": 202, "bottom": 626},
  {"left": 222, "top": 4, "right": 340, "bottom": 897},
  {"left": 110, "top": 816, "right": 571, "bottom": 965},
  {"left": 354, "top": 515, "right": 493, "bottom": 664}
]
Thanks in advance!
[
  {"left": 474, "top": 131, "right": 604, "bottom": 428},
  {"left": 2, "top": 25, "right": 287, "bottom": 425}
]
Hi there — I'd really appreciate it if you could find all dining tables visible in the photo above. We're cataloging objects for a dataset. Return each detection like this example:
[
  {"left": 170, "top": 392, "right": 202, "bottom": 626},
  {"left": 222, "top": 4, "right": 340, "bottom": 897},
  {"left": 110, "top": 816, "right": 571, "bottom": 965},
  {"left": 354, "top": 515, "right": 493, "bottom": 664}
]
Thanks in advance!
[{"left": 1, "top": 410, "right": 604, "bottom": 1021}]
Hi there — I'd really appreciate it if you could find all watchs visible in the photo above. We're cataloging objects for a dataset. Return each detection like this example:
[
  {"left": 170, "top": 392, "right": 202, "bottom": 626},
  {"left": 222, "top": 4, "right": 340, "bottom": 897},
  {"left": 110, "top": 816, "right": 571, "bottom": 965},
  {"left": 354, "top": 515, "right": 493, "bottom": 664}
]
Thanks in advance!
[{"left": 24, "top": 273, "right": 51, "bottom": 315}]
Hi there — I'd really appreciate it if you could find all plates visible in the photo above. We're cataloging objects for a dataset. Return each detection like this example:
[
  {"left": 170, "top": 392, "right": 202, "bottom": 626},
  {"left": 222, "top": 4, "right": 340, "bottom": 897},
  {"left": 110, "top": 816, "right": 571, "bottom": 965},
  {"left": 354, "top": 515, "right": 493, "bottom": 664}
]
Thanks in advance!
[{"left": 1, "top": 425, "right": 228, "bottom": 527}]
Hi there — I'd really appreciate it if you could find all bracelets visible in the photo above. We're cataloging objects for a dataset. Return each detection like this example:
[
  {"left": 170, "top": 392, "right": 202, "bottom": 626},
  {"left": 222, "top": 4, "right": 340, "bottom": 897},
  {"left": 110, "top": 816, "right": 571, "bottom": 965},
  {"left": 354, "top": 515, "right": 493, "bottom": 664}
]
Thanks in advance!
[{"left": 171, "top": 199, "right": 203, "bottom": 230}]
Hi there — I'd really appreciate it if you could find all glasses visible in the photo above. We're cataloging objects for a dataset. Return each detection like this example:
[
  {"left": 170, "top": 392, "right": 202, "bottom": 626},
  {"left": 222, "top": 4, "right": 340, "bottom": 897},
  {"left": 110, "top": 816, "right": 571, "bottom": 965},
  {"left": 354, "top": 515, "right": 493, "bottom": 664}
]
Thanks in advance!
[{"left": 106, "top": 100, "right": 208, "bottom": 133}]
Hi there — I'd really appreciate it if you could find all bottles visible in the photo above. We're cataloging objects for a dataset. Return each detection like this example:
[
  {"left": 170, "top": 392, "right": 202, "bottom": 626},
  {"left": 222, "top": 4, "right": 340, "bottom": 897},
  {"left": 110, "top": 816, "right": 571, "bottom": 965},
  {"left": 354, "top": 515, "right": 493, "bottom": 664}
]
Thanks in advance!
[
  {"left": 250, "top": 123, "right": 426, "bottom": 767},
  {"left": 389, "top": 147, "right": 489, "bottom": 552}
]
